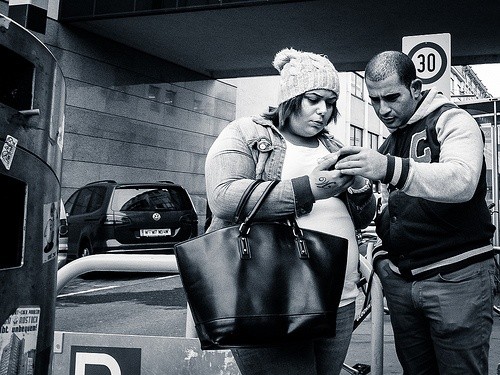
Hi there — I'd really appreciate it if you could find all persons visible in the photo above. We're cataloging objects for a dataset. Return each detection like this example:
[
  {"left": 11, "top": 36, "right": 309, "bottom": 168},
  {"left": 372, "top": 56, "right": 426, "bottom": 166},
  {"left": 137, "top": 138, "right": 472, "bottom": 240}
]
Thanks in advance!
[
  {"left": 334, "top": 51, "right": 498, "bottom": 375},
  {"left": 204, "top": 47, "right": 377, "bottom": 375}
]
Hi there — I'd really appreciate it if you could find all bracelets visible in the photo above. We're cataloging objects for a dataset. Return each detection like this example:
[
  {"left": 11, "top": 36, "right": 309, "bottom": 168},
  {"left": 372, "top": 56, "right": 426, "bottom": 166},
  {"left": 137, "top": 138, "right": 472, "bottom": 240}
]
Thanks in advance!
[{"left": 346, "top": 175, "right": 371, "bottom": 195}]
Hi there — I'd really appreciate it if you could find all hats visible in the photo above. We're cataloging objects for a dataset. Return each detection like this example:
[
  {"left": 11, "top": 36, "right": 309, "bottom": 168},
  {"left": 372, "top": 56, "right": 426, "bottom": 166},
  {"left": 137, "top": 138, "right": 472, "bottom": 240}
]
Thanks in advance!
[{"left": 273, "top": 47, "right": 340, "bottom": 106}]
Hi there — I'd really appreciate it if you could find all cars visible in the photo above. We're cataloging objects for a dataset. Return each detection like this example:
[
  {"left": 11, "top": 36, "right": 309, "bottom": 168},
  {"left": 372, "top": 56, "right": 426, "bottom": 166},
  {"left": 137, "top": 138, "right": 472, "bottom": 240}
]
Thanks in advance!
[{"left": 360, "top": 218, "right": 378, "bottom": 239}]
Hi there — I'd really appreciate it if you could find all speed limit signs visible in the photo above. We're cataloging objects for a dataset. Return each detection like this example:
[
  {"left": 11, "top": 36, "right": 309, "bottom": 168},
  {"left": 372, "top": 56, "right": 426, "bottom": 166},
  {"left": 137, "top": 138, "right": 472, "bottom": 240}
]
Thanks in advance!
[{"left": 403, "top": 33, "right": 452, "bottom": 97}]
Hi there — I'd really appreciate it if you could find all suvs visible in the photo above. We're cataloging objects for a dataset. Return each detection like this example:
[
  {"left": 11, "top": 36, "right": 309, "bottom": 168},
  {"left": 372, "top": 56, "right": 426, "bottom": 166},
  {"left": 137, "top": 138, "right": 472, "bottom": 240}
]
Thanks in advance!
[{"left": 60, "top": 179, "right": 199, "bottom": 282}]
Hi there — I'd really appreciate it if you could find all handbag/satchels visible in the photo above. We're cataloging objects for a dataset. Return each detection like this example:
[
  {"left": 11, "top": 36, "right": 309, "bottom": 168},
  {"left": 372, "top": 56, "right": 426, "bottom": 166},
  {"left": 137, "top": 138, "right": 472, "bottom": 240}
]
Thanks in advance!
[{"left": 175, "top": 179, "right": 349, "bottom": 350}]
